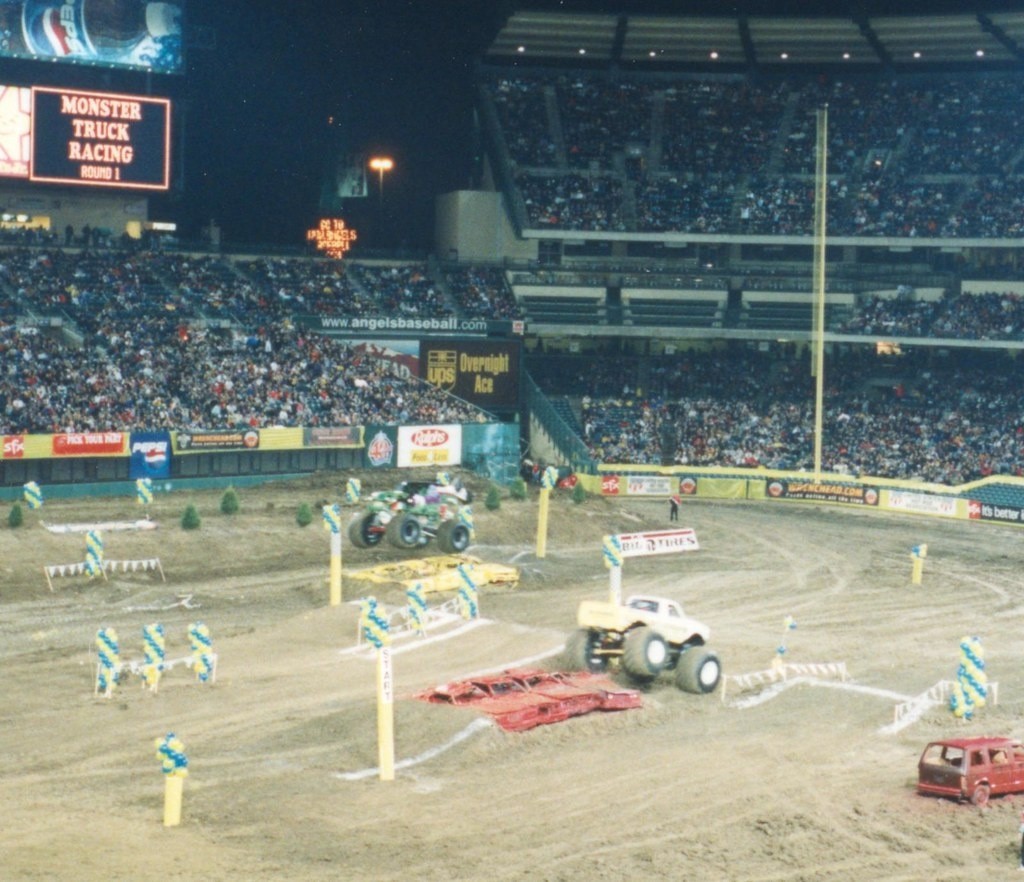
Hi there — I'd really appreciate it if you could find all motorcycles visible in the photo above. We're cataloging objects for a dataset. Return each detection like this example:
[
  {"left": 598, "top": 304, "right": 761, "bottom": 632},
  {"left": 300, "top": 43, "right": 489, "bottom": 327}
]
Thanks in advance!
[
  {"left": 347, "top": 475, "right": 479, "bottom": 557},
  {"left": 576, "top": 595, "right": 722, "bottom": 695}
]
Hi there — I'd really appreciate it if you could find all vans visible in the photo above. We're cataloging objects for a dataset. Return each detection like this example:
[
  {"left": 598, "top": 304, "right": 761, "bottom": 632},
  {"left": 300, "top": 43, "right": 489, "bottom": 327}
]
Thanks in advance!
[{"left": 916, "top": 738, "right": 1023, "bottom": 807}]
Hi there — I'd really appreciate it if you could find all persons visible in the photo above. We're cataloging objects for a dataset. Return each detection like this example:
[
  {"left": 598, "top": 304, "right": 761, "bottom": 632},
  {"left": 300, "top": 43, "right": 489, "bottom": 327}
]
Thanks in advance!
[
  {"left": 669, "top": 493, "right": 680, "bottom": 522},
  {"left": 530, "top": 344, "right": 1024, "bottom": 486},
  {"left": 851, "top": 289, "right": 1024, "bottom": 341},
  {"left": 0, "top": 222, "right": 527, "bottom": 435},
  {"left": 514, "top": 248, "right": 1024, "bottom": 294},
  {"left": 495, "top": 65, "right": 1024, "bottom": 237}
]
